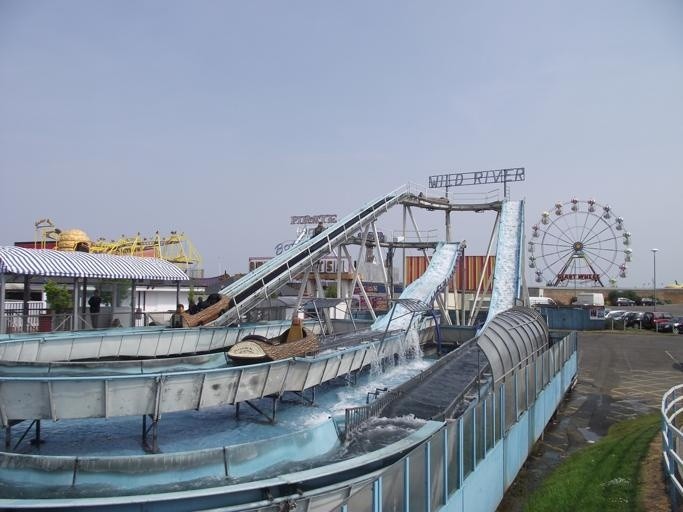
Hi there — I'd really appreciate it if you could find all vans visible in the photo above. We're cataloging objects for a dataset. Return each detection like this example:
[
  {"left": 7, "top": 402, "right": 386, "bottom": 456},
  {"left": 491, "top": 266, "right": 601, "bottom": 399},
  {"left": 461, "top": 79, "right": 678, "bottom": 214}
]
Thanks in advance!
[{"left": 646, "top": 311, "right": 673, "bottom": 331}]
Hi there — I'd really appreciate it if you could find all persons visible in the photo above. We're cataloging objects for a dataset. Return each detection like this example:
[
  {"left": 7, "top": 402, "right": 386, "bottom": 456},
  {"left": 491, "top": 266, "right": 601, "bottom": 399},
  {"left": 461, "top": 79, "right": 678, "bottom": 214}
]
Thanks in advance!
[
  {"left": 188, "top": 293, "right": 219, "bottom": 316},
  {"left": 314, "top": 221, "right": 325, "bottom": 235},
  {"left": 111, "top": 318, "right": 122, "bottom": 327},
  {"left": 171, "top": 304, "right": 184, "bottom": 327},
  {"left": 87, "top": 289, "right": 100, "bottom": 328}
]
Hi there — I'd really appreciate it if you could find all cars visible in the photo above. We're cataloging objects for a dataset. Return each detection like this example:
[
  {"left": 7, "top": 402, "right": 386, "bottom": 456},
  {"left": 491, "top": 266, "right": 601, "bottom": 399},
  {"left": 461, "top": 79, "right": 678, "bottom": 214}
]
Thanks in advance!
[
  {"left": 636, "top": 298, "right": 657, "bottom": 306},
  {"left": 668, "top": 316, "right": 683, "bottom": 334},
  {"left": 604, "top": 308, "right": 644, "bottom": 321},
  {"left": 615, "top": 297, "right": 635, "bottom": 307}
]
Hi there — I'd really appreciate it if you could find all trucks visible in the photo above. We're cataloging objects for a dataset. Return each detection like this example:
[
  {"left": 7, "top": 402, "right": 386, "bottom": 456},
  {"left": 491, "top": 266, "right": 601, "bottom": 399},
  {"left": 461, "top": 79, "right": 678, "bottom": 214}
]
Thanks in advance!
[{"left": 528, "top": 297, "right": 557, "bottom": 306}]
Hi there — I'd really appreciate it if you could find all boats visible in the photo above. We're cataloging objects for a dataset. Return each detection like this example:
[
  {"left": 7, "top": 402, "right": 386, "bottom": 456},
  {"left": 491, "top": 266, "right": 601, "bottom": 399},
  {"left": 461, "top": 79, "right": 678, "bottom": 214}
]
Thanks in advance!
[
  {"left": 226, "top": 317, "right": 320, "bottom": 363},
  {"left": 171, "top": 293, "right": 229, "bottom": 327}
]
